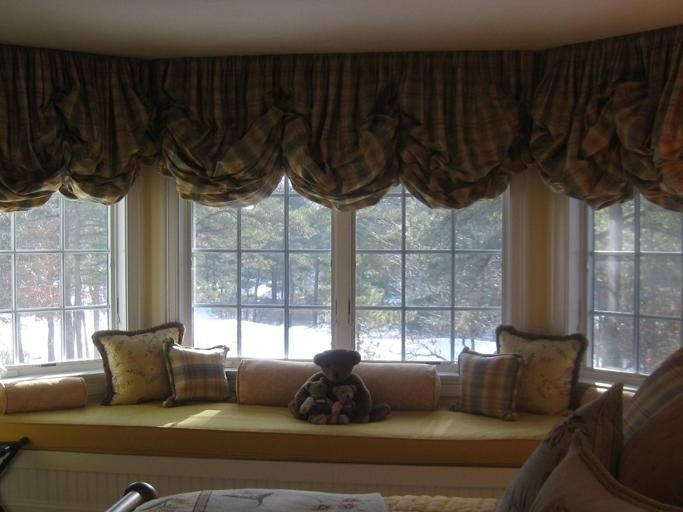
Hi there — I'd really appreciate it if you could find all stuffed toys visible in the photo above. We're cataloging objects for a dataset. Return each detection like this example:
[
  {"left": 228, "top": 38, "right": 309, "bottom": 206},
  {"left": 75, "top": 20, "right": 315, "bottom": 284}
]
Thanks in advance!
[
  {"left": 299, "top": 376, "right": 350, "bottom": 425},
  {"left": 287, "top": 349, "right": 390, "bottom": 422},
  {"left": 332, "top": 384, "right": 371, "bottom": 426}
]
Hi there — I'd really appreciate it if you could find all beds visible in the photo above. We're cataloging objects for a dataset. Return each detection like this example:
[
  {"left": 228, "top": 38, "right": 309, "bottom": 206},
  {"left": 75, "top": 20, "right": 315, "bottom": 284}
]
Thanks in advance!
[{"left": 130, "top": 487, "right": 501, "bottom": 511}]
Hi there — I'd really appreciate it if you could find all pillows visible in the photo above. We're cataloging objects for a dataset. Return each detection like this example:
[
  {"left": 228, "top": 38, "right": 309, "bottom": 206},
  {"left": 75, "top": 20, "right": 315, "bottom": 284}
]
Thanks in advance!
[
  {"left": 450, "top": 347, "right": 523, "bottom": 421},
  {"left": 163, "top": 338, "right": 236, "bottom": 406},
  {"left": 91, "top": 321, "right": 185, "bottom": 406},
  {"left": 496, "top": 325, "right": 588, "bottom": 415},
  {"left": 529, "top": 430, "right": 683, "bottom": 512},
  {"left": 498, "top": 384, "right": 624, "bottom": 511},
  {"left": 236, "top": 358, "right": 441, "bottom": 410},
  {"left": 0, "top": 377, "right": 88, "bottom": 415},
  {"left": 618, "top": 346, "right": 683, "bottom": 507}
]
